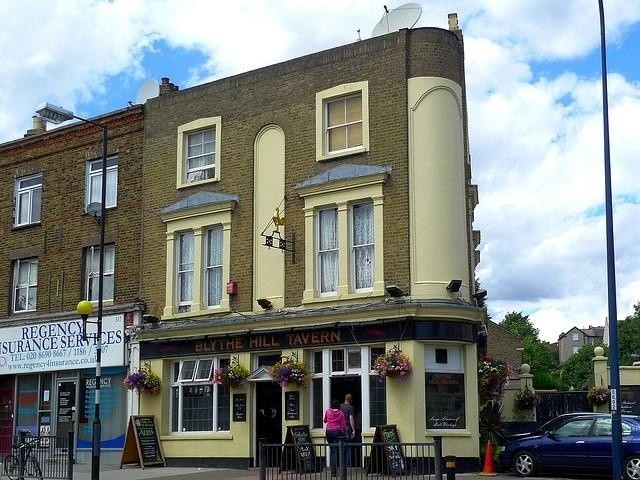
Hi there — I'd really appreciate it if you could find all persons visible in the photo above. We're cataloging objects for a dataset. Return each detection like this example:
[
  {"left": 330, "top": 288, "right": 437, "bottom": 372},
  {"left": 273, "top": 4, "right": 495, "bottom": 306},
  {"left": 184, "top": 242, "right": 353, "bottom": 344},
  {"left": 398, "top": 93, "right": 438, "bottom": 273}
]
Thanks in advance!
[
  {"left": 340, "top": 393, "right": 356, "bottom": 467},
  {"left": 324, "top": 399, "right": 346, "bottom": 477}
]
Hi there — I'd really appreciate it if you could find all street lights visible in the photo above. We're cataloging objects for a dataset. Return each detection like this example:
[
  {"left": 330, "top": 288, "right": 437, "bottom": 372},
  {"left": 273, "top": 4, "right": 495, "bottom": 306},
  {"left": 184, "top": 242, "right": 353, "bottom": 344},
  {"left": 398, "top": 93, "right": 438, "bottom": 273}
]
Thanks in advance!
[{"left": 33, "top": 101, "right": 109, "bottom": 479}]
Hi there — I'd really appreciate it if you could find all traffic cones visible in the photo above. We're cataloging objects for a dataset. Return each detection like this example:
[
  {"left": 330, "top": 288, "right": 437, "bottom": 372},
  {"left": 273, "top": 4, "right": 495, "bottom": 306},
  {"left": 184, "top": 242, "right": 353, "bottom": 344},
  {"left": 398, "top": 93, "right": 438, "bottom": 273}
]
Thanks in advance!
[{"left": 478, "top": 437, "right": 499, "bottom": 478}]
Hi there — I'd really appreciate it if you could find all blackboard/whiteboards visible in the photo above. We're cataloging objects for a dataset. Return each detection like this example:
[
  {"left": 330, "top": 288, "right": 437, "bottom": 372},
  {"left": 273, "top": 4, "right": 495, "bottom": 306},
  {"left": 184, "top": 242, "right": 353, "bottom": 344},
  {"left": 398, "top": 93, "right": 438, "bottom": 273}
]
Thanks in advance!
[
  {"left": 285, "top": 390, "right": 300, "bottom": 421},
  {"left": 131, "top": 414, "right": 166, "bottom": 466},
  {"left": 289, "top": 425, "right": 314, "bottom": 474},
  {"left": 378, "top": 424, "right": 408, "bottom": 476},
  {"left": 231, "top": 392, "right": 248, "bottom": 423}
]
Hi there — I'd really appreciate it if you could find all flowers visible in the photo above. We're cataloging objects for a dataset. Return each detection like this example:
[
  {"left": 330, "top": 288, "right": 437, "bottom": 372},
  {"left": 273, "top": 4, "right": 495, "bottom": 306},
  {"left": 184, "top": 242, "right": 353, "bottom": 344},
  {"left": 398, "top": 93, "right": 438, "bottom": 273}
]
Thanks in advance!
[
  {"left": 477, "top": 354, "right": 513, "bottom": 403},
  {"left": 373, "top": 345, "right": 412, "bottom": 384},
  {"left": 210, "top": 364, "right": 252, "bottom": 387},
  {"left": 122, "top": 370, "right": 162, "bottom": 394},
  {"left": 514, "top": 387, "right": 544, "bottom": 409},
  {"left": 588, "top": 386, "right": 612, "bottom": 407},
  {"left": 271, "top": 355, "right": 310, "bottom": 391}
]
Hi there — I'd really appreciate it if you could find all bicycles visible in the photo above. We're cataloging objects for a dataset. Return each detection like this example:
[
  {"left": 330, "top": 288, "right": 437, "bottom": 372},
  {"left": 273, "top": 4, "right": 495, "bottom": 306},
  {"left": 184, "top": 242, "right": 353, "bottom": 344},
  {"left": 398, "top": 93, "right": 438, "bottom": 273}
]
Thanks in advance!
[{"left": 4, "top": 431, "right": 45, "bottom": 480}]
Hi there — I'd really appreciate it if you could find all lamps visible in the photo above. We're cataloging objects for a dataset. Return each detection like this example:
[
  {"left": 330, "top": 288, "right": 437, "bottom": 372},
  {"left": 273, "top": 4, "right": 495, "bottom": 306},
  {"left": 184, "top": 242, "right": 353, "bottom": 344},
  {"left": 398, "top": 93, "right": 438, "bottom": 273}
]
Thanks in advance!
[
  {"left": 472, "top": 291, "right": 488, "bottom": 300},
  {"left": 257, "top": 299, "right": 273, "bottom": 309},
  {"left": 143, "top": 314, "right": 160, "bottom": 324},
  {"left": 387, "top": 284, "right": 403, "bottom": 297},
  {"left": 448, "top": 280, "right": 463, "bottom": 293}
]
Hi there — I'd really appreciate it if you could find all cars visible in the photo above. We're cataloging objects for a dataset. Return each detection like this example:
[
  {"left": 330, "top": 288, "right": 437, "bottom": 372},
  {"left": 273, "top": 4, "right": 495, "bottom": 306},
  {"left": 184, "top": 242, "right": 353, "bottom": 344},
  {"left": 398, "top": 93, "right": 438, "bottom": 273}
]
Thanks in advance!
[{"left": 500, "top": 407, "right": 640, "bottom": 480}]
